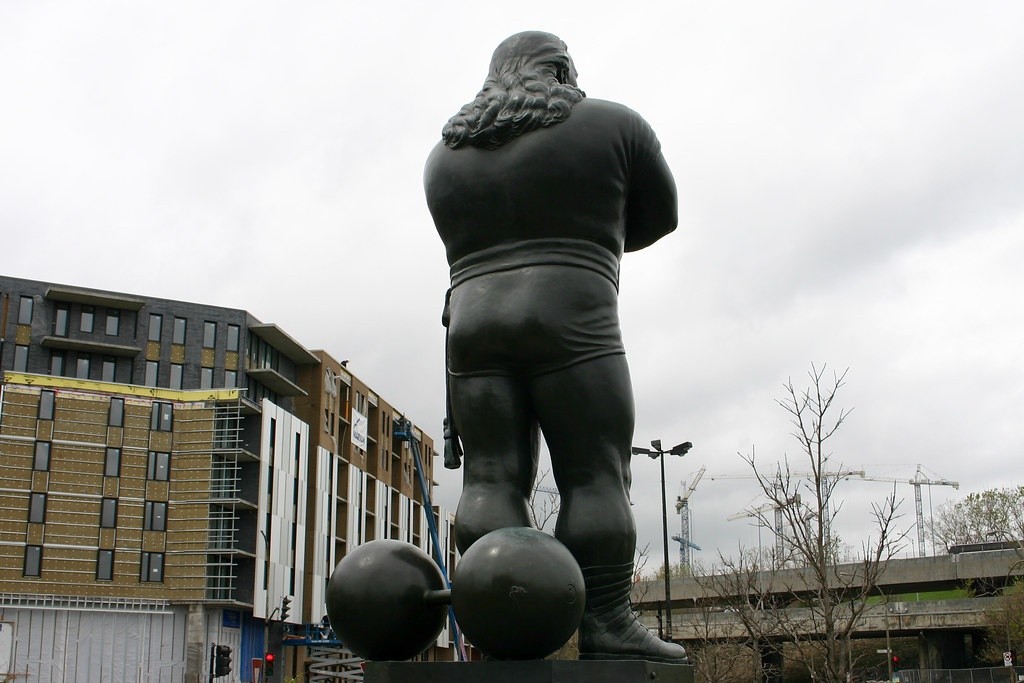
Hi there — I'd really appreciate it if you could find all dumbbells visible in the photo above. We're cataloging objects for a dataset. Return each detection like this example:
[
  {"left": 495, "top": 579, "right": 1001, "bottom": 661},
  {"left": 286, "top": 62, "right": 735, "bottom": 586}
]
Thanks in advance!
[{"left": 324, "top": 527, "right": 587, "bottom": 657}]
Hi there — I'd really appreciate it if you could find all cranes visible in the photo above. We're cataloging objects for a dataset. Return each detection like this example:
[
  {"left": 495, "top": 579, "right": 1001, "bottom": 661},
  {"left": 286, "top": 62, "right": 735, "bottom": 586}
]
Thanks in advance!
[
  {"left": 670, "top": 464, "right": 707, "bottom": 578},
  {"left": 727, "top": 485, "right": 801, "bottom": 571},
  {"left": 771, "top": 460, "right": 866, "bottom": 568},
  {"left": 864, "top": 465, "right": 959, "bottom": 558}
]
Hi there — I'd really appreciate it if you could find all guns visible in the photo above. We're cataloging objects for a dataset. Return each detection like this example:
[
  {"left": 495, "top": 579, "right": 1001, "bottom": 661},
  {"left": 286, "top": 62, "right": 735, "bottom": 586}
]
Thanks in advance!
[{"left": 443, "top": 288, "right": 467, "bottom": 467}]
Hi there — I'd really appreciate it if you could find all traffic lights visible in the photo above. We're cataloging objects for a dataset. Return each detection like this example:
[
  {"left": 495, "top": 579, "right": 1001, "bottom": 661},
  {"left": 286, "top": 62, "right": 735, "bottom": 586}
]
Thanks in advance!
[
  {"left": 281, "top": 596, "right": 292, "bottom": 621},
  {"left": 216, "top": 645, "right": 233, "bottom": 677},
  {"left": 264, "top": 651, "right": 275, "bottom": 678}
]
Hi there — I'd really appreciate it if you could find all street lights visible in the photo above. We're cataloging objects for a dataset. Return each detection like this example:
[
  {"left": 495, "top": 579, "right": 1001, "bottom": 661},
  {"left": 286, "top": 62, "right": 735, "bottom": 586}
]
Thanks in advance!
[{"left": 631, "top": 439, "right": 693, "bottom": 641}]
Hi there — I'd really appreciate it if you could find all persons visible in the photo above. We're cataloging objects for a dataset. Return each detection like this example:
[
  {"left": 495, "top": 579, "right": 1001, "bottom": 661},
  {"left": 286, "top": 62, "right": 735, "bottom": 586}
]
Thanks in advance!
[{"left": 423, "top": 31, "right": 692, "bottom": 665}]
